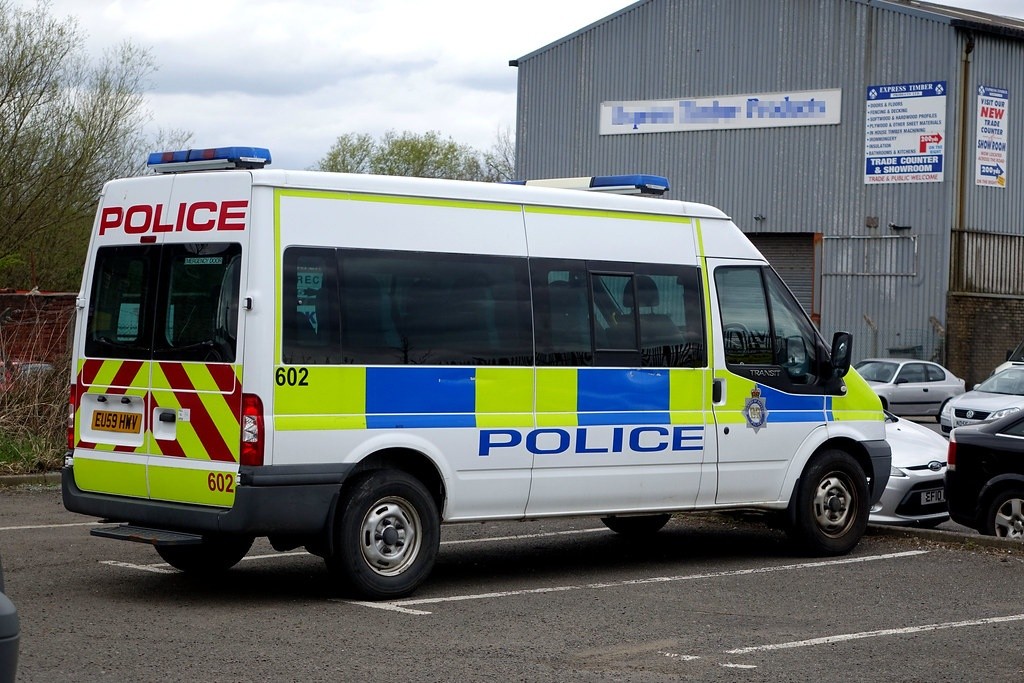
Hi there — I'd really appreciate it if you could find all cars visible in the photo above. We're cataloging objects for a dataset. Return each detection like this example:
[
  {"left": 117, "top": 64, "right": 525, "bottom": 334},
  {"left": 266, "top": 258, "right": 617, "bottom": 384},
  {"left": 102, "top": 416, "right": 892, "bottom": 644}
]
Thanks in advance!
[
  {"left": 868, "top": 408, "right": 951, "bottom": 529},
  {"left": 989, "top": 339, "right": 1024, "bottom": 391},
  {"left": 940, "top": 366, "right": 1024, "bottom": 438},
  {"left": 0, "top": 361, "right": 56, "bottom": 400},
  {"left": 944, "top": 407, "right": 1023, "bottom": 540},
  {"left": 853, "top": 357, "right": 966, "bottom": 422}
]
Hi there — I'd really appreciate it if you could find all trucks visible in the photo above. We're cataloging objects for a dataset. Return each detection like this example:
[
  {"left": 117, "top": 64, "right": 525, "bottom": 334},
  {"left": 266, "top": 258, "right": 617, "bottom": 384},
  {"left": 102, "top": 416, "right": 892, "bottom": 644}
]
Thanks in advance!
[{"left": 61, "top": 145, "right": 892, "bottom": 598}]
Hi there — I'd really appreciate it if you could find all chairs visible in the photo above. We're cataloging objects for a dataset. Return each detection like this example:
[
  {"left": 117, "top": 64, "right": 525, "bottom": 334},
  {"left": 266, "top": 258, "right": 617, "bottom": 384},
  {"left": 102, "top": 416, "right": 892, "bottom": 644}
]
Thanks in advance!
[
  {"left": 541, "top": 279, "right": 604, "bottom": 346},
  {"left": 609, "top": 275, "right": 685, "bottom": 347}
]
